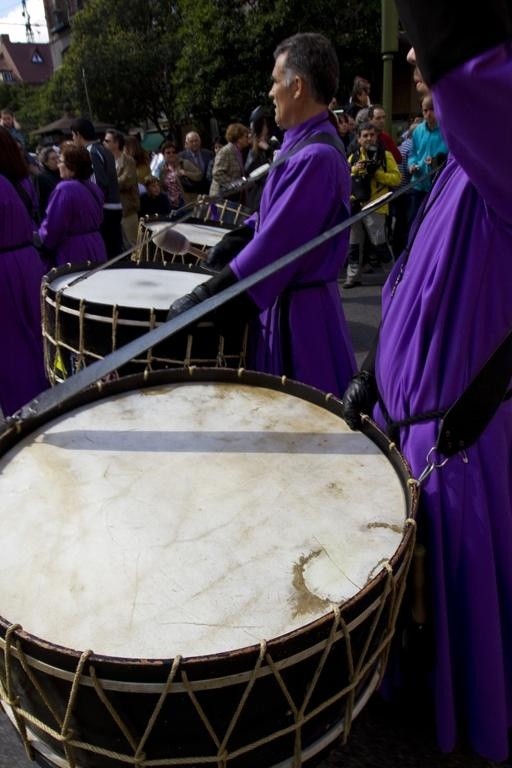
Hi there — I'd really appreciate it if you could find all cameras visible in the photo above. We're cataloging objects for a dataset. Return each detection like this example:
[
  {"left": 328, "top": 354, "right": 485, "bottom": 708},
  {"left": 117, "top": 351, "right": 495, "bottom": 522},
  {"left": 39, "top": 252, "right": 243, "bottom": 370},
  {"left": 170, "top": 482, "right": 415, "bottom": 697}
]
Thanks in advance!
[{"left": 365, "top": 160, "right": 377, "bottom": 176}]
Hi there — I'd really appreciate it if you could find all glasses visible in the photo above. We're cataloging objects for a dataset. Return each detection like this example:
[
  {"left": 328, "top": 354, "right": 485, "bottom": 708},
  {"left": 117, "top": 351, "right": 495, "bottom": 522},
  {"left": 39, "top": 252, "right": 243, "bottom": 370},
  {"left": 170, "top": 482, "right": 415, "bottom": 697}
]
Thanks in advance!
[{"left": 166, "top": 152, "right": 177, "bottom": 154}]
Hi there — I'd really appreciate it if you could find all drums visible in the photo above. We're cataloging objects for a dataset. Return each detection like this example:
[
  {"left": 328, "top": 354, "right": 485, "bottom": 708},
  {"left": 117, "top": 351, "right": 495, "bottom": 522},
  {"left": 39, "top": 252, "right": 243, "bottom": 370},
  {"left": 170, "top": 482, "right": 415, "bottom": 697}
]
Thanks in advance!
[
  {"left": 130, "top": 194, "right": 255, "bottom": 270},
  {"left": 41, "top": 261, "right": 250, "bottom": 390},
  {"left": 0, "top": 365, "right": 420, "bottom": 768}
]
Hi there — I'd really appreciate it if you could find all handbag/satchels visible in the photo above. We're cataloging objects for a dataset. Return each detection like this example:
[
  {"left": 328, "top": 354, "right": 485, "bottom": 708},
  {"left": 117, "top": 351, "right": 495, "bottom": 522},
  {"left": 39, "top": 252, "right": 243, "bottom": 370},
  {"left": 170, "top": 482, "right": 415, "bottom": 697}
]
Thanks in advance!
[{"left": 180, "top": 174, "right": 211, "bottom": 194}]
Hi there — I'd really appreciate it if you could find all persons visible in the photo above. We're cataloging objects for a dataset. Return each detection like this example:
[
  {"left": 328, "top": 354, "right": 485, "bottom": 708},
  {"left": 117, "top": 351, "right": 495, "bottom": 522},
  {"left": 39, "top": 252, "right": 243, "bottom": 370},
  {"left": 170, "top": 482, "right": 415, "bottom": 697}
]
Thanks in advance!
[
  {"left": 32, "top": 143, "right": 110, "bottom": 270},
  {"left": 325, "top": 79, "right": 451, "bottom": 290},
  {"left": 2, "top": 129, "right": 48, "bottom": 418},
  {"left": 166, "top": 32, "right": 359, "bottom": 398},
  {"left": 2, "top": 103, "right": 283, "bottom": 249},
  {"left": 345, "top": 0, "right": 511, "bottom": 768}
]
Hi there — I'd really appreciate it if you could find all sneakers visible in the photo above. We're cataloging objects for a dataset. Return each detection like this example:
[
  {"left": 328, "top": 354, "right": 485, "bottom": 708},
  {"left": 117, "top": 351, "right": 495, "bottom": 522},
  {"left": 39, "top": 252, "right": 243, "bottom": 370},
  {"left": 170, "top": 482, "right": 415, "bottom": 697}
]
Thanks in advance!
[{"left": 343, "top": 281, "right": 361, "bottom": 288}]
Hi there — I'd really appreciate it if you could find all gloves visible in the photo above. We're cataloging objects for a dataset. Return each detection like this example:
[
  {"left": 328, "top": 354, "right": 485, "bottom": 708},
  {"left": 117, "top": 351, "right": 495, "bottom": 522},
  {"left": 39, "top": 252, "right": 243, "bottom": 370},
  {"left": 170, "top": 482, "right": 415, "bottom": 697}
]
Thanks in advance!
[
  {"left": 201, "top": 225, "right": 255, "bottom": 271},
  {"left": 166, "top": 269, "right": 239, "bottom": 321}
]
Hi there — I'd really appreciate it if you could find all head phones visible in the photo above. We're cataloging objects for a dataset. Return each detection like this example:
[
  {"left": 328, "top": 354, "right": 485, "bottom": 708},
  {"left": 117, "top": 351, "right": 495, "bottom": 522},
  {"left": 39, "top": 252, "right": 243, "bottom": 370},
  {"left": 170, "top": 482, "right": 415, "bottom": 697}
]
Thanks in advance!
[{"left": 368, "top": 107, "right": 373, "bottom": 117}]
